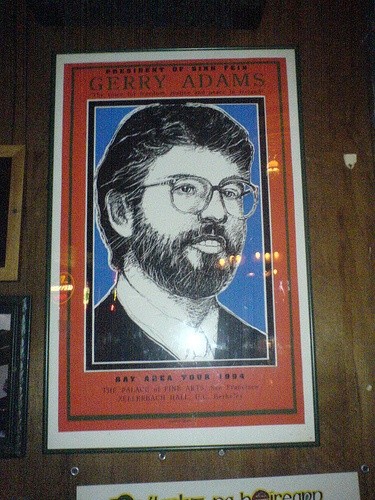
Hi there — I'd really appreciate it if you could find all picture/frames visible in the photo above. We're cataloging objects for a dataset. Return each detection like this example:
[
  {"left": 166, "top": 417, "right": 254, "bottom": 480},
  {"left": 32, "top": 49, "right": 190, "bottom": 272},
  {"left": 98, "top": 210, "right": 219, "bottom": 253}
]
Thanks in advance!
[
  {"left": 0, "top": 144, "right": 27, "bottom": 281},
  {"left": 43, "top": 46, "right": 322, "bottom": 455},
  {"left": 0, "top": 294, "right": 32, "bottom": 458}
]
